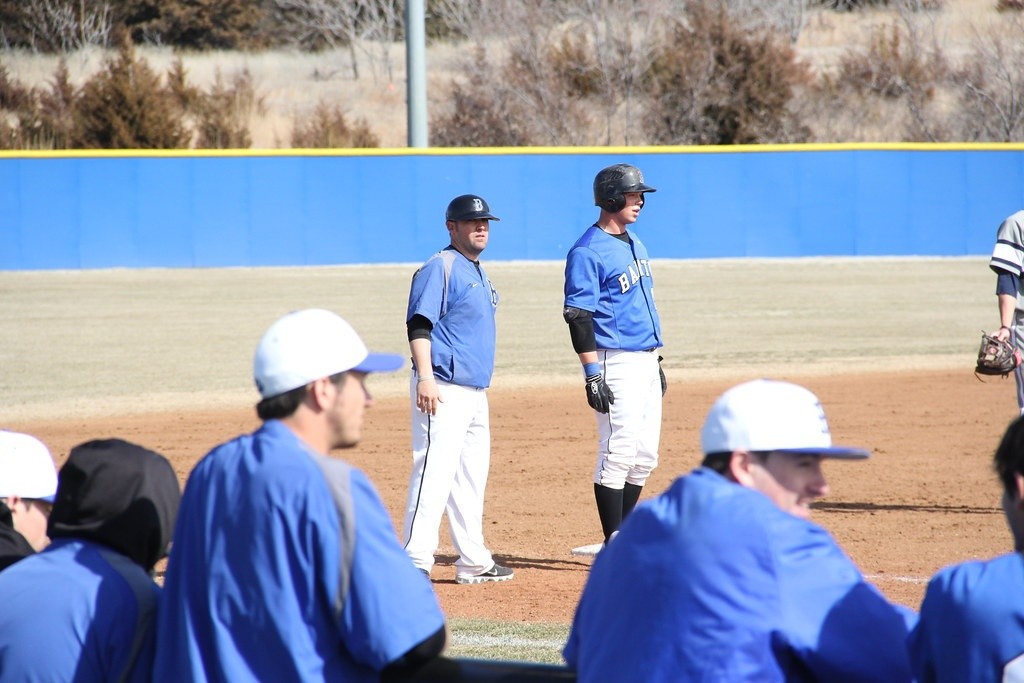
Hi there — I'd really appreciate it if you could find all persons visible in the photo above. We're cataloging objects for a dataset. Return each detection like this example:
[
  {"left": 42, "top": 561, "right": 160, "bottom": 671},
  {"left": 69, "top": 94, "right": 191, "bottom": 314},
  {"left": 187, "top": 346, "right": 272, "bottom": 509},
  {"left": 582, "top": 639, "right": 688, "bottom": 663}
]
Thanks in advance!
[
  {"left": 151, "top": 307, "right": 461, "bottom": 683},
  {"left": 0, "top": 438, "right": 181, "bottom": 683},
  {"left": 562, "top": 163, "right": 667, "bottom": 551},
  {"left": 401, "top": 194, "right": 514, "bottom": 584},
  {"left": 989, "top": 209, "right": 1024, "bottom": 414},
  {"left": 561, "top": 377, "right": 935, "bottom": 683},
  {"left": 919, "top": 415, "right": 1024, "bottom": 683},
  {"left": 0, "top": 429, "right": 58, "bottom": 574}
]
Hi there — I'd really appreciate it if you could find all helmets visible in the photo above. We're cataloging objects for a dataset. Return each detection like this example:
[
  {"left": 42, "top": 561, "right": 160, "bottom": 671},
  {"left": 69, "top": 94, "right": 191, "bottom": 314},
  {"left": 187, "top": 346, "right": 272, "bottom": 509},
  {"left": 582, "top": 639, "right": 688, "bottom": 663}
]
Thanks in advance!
[{"left": 594, "top": 164, "right": 656, "bottom": 213}]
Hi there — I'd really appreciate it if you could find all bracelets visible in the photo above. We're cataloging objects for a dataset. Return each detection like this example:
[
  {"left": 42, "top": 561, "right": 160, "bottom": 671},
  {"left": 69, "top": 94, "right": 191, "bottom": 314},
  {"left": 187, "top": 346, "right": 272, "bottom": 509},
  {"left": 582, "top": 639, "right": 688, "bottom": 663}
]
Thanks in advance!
[
  {"left": 417, "top": 376, "right": 434, "bottom": 382},
  {"left": 1001, "top": 326, "right": 1011, "bottom": 333}
]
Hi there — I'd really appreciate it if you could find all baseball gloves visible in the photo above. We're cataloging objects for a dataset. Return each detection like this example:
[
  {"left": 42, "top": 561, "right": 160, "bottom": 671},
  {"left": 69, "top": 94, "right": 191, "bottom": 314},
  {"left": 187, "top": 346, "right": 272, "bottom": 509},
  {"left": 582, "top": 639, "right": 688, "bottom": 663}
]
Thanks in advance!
[{"left": 974, "top": 332, "right": 1023, "bottom": 375}]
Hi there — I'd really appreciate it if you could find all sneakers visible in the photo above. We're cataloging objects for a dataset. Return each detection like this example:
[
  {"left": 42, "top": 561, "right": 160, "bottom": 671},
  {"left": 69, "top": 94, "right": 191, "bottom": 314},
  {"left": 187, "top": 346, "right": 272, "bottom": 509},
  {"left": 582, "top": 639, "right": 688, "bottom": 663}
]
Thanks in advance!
[{"left": 456, "top": 564, "right": 514, "bottom": 584}]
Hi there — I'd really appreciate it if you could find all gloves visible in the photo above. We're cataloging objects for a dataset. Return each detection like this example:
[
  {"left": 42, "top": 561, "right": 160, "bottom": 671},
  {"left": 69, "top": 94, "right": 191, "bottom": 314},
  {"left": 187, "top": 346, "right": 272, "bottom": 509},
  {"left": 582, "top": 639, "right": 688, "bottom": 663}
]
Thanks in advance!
[
  {"left": 658, "top": 356, "right": 667, "bottom": 397},
  {"left": 585, "top": 373, "right": 614, "bottom": 414}
]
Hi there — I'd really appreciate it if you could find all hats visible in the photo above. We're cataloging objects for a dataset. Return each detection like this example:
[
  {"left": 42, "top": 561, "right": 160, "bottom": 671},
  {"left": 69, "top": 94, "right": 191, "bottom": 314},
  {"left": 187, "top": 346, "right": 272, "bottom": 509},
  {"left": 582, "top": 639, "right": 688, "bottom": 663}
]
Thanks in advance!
[
  {"left": 0, "top": 429, "right": 58, "bottom": 503},
  {"left": 446, "top": 194, "right": 500, "bottom": 221},
  {"left": 701, "top": 379, "right": 870, "bottom": 459},
  {"left": 254, "top": 308, "right": 402, "bottom": 400}
]
